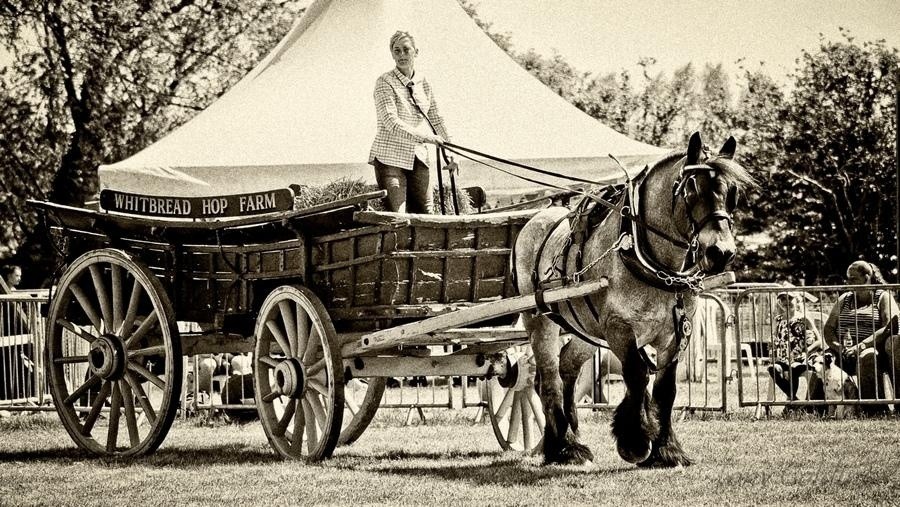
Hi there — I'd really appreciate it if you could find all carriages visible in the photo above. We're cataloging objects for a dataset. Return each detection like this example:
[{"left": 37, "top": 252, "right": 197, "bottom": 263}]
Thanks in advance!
[{"left": 24, "top": 129, "right": 762, "bottom": 469}]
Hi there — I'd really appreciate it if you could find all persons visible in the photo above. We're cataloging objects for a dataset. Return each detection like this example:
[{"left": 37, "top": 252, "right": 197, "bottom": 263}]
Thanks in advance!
[
  {"left": 764, "top": 283, "right": 828, "bottom": 420},
  {"left": 366, "top": 28, "right": 461, "bottom": 215},
  {"left": 2, "top": 263, "right": 23, "bottom": 292},
  {"left": 823, "top": 259, "right": 900, "bottom": 419}
]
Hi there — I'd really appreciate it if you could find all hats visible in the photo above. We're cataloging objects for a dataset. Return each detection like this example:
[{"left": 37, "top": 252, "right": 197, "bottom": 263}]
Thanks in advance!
[{"left": 775, "top": 282, "right": 798, "bottom": 299}]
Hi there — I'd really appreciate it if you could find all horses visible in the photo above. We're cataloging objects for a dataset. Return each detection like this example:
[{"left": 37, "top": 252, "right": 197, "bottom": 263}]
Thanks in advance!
[{"left": 512, "top": 129, "right": 760, "bottom": 471}]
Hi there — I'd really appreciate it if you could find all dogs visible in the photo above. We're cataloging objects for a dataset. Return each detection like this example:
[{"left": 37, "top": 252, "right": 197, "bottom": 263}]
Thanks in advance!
[{"left": 807, "top": 351, "right": 859, "bottom": 421}]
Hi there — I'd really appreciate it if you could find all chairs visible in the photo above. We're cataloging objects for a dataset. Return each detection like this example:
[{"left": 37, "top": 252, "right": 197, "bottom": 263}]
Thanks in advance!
[{"left": 690, "top": 293, "right": 829, "bottom": 415}]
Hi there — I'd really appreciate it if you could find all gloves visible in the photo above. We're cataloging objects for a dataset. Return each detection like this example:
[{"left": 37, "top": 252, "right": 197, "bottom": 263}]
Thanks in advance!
[{"left": 424, "top": 134, "right": 446, "bottom": 149}]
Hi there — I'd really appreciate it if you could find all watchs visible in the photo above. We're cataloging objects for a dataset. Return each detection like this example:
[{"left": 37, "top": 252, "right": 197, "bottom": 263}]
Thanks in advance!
[{"left": 859, "top": 341, "right": 866, "bottom": 351}]
